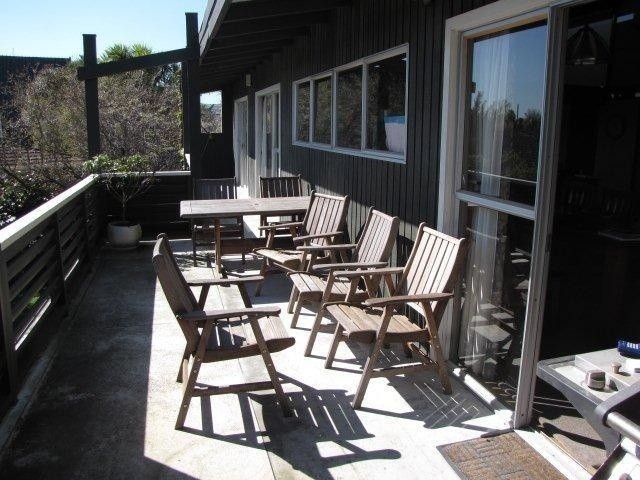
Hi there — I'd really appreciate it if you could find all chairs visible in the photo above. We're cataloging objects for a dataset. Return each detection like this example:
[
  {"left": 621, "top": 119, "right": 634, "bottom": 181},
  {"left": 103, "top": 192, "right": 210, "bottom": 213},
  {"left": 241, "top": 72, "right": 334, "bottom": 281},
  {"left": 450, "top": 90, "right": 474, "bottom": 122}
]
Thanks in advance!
[
  {"left": 151, "top": 232, "right": 298, "bottom": 432},
  {"left": 258, "top": 172, "right": 305, "bottom": 255},
  {"left": 463, "top": 222, "right": 532, "bottom": 388},
  {"left": 285, "top": 204, "right": 403, "bottom": 358},
  {"left": 189, "top": 176, "right": 249, "bottom": 267},
  {"left": 251, "top": 187, "right": 352, "bottom": 316},
  {"left": 318, "top": 220, "right": 471, "bottom": 411}
]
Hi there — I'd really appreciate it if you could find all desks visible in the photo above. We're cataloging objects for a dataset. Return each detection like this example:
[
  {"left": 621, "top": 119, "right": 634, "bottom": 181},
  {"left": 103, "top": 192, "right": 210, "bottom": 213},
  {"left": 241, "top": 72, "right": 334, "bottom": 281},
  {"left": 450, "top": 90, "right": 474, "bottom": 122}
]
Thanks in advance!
[
  {"left": 179, "top": 195, "right": 314, "bottom": 274},
  {"left": 533, "top": 340, "right": 640, "bottom": 463}
]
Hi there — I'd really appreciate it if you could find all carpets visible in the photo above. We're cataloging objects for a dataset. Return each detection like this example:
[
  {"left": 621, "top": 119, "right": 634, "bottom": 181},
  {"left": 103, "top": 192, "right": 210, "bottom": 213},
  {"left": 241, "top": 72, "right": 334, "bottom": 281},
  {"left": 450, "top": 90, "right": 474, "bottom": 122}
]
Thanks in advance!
[{"left": 435, "top": 425, "right": 572, "bottom": 480}]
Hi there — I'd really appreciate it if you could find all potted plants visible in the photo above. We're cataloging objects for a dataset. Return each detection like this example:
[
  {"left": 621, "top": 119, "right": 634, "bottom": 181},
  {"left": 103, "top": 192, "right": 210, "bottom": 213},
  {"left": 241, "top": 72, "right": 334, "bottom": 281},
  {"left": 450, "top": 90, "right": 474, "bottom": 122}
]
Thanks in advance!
[{"left": 80, "top": 151, "right": 163, "bottom": 252}]
